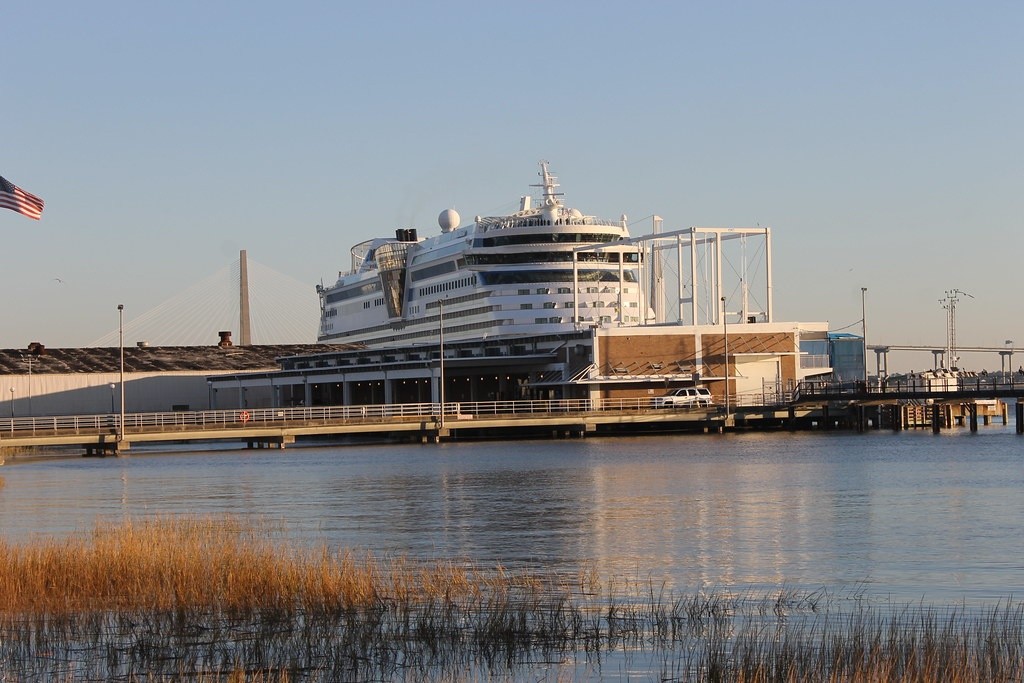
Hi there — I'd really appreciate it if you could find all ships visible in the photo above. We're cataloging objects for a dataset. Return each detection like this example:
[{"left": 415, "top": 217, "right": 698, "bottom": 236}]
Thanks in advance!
[{"left": 276, "top": 156, "right": 659, "bottom": 405}]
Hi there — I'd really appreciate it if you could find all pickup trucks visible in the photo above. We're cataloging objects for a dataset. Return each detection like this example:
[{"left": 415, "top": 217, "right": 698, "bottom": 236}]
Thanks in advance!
[{"left": 650, "top": 388, "right": 713, "bottom": 408}]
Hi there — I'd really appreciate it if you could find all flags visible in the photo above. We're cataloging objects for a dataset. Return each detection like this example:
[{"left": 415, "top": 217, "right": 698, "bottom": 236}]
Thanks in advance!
[{"left": 0, "top": 176, "right": 44, "bottom": 220}]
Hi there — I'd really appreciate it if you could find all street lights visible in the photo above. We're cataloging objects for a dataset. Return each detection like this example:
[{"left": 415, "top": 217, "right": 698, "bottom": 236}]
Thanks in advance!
[
  {"left": 117, "top": 304, "right": 126, "bottom": 440},
  {"left": 861, "top": 287, "right": 867, "bottom": 385},
  {"left": 436, "top": 298, "right": 446, "bottom": 428},
  {"left": 109, "top": 383, "right": 115, "bottom": 413},
  {"left": 207, "top": 381, "right": 212, "bottom": 410},
  {"left": 10, "top": 386, "right": 15, "bottom": 417},
  {"left": 721, "top": 295, "right": 730, "bottom": 416},
  {"left": 20, "top": 354, "right": 40, "bottom": 416}
]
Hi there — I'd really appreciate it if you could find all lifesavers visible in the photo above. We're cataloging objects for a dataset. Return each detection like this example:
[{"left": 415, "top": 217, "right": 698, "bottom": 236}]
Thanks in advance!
[{"left": 239, "top": 411, "right": 250, "bottom": 422}]
